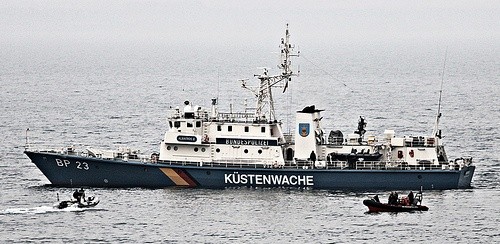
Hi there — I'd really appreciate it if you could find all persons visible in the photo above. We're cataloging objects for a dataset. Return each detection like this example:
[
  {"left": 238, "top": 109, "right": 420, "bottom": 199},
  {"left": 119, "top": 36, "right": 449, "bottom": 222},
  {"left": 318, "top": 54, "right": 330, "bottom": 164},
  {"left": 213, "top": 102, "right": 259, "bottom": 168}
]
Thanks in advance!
[
  {"left": 408, "top": 190, "right": 414, "bottom": 206},
  {"left": 310, "top": 150, "right": 316, "bottom": 161},
  {"left": 373, "top": 195, "right": 380, "bottom": 203},
  {"left": 388, "top": 192, "right": 399, "bottom": 204},
  {"left": 79, "top": 187, "right": 86, "bottom": 203},
  {"left": 73, "top": 189, "right": 80, "bottom": 202}
]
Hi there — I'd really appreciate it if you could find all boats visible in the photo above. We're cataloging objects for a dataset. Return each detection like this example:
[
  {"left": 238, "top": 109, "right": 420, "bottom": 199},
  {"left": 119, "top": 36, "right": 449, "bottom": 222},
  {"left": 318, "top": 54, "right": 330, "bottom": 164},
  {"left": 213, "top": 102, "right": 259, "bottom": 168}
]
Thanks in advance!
[
  {"left": 52, "top": 195, "right": 99, "bottom": 209},
  {"left": 363, "top": 190, "right": 429, "bottom": 212}
]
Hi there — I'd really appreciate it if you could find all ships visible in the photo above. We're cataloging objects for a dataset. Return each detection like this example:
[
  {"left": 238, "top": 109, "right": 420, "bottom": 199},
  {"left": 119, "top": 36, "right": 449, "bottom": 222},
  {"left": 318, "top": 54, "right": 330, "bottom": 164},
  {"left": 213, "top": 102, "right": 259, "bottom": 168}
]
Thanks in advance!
[{"left": 24, "top": 24, "right": 477, "bottom": 190}]
